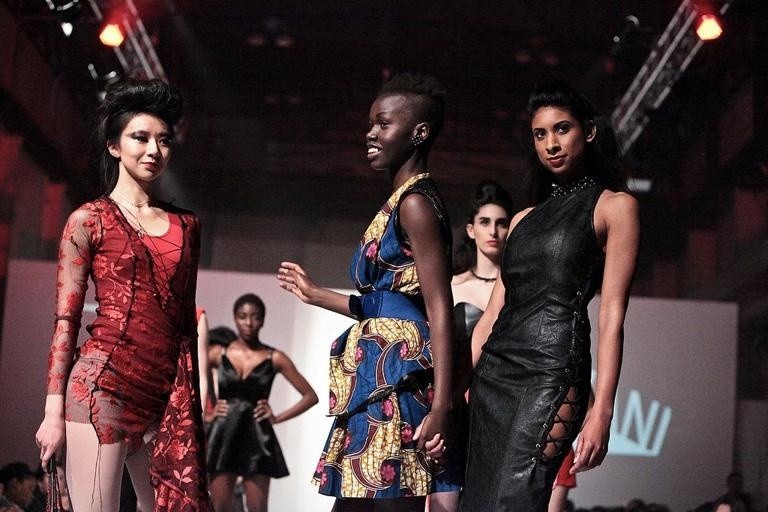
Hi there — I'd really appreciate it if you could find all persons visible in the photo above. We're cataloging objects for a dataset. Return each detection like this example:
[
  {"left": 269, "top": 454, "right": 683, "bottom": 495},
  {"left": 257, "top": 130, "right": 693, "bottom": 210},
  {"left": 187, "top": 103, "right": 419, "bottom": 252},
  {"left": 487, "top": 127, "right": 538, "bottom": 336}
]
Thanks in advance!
[
  {"left": 207, "top": 292, "right": 319, "bottom": 512},
  {"left": 411, "top": 88, "right": 641, "bottom": 512},
  {"left": 447, "top": 181, "right": 519, "bottom": 366},
  {"left": 716, "top": 472, "right": 752, "bottom": 507},
  {"left": 277, "top": 67, "right": 454, "bottom": 512},
  {"left": 0, "top": 461, "right": 39, "bottom": 512},
  {"left": 26, "top": 454, "right": 74, "bottom": 512},
  {"left": 561, "top": 493, "right": 766, "bottom": 512},
  {"left": 33, "top": 76, "right": 214, "bottom": 512},
  {"left": 204, "top": 327, "right": 238, "bottom": 418}
]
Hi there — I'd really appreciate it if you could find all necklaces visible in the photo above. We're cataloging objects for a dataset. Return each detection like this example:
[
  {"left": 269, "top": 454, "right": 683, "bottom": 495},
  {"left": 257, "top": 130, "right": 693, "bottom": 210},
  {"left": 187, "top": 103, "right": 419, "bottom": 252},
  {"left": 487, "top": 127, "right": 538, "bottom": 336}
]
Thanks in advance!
[
  {"left": 470, "top": 266, "right": 497, "bottom": 283},
  {"left": 111, "top": 188, "right": 152, "bottom": 240}
]
